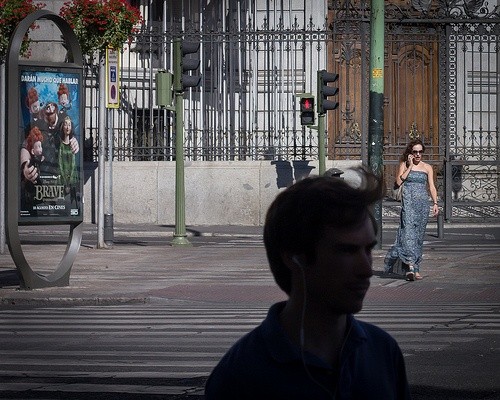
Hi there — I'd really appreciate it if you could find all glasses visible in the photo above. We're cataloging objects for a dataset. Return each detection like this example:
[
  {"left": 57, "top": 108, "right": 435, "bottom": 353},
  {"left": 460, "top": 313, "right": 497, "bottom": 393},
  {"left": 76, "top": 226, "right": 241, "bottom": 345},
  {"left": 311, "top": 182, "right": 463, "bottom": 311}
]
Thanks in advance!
[{"left": 412, "top": 150, "right": 423, "bottom": 154}]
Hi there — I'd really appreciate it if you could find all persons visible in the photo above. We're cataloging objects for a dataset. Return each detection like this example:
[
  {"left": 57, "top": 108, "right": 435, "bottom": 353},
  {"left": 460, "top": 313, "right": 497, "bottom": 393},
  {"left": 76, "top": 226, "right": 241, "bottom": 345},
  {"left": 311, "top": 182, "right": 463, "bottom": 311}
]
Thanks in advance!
[
  {"left": 384, "top": 139, "right": 439, "bottom": 281},
  {"left": 204, "top": 163, "right": 409, "bottom": 400}
]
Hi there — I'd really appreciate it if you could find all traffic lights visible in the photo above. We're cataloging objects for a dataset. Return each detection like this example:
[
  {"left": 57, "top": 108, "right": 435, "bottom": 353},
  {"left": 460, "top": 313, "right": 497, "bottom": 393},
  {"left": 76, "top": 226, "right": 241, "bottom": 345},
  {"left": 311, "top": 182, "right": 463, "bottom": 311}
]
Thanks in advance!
[
  {"left": 299, "top": 94, "right": 314, "bottom": 126},
  {"left": 173, "top": 37, "right": 202, "bottom": 91},
  {"left": 317, "top": 69, "right": 339, "bottom": 114}
]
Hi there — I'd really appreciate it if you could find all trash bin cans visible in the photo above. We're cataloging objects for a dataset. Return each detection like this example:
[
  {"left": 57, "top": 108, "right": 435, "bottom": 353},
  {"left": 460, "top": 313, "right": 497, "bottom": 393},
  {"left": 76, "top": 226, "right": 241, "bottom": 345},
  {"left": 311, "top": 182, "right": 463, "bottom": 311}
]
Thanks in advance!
[{"left": 104, "top": 213, "right": 114, "bottom": 246}]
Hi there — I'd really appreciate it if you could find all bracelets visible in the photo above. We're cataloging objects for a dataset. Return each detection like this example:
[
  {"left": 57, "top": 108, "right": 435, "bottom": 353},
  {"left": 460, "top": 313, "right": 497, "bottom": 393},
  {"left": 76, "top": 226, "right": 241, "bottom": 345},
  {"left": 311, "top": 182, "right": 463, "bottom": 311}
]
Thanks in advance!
[
  {"left": 400, "top": 175, "right": 405, "bottom": 182},
  {"left": 434, "top": 203, "right": 438, "bottom": 205}
]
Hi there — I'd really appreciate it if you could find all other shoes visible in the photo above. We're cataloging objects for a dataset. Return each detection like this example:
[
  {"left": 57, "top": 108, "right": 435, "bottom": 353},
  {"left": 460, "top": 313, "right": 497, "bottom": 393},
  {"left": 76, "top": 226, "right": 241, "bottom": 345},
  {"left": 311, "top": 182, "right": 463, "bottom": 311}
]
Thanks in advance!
[
  {"left": 406, "top": 270, "right": 415, "bottom": 281},
  {"left": 414, "top": 273, "right": 422, "bottom": 280}
]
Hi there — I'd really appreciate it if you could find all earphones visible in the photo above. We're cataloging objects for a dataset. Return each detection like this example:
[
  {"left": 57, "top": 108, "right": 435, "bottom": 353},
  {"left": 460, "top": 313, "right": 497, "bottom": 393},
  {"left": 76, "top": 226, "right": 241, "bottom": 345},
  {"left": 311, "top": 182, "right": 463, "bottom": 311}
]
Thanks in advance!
[{"left": 291, "top": 255, "right": 302, "bottom": 269}]
